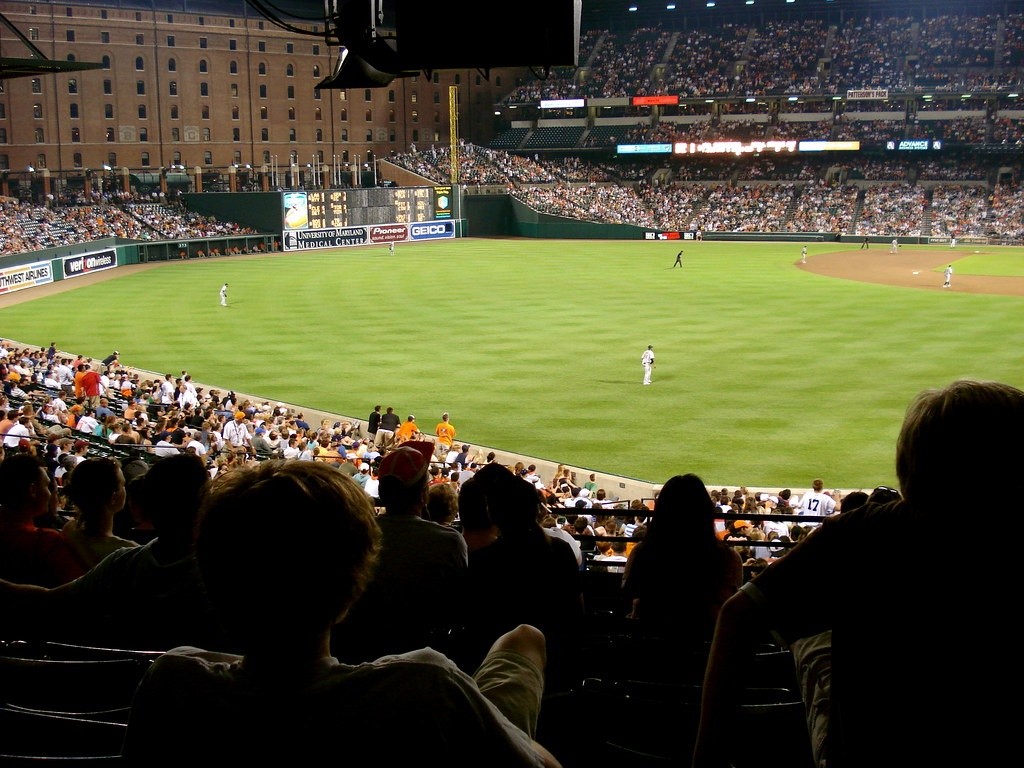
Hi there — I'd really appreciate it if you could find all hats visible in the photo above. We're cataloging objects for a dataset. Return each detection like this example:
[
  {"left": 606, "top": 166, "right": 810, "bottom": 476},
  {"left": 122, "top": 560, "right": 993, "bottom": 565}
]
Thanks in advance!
[
  {"left": 376, "top": 440, "right": 434, "bottom": 483},
  {"left": 734, "top": 519, "right": 750, "bottom": 529},
  {"left": 255, "top": 428, "right": 265, "bottom": 434},
  {"left": 791, "top": 526, "right": 802, "bottom": 541},
  {"left": 760, "top": 494, "right": 769, "bottom": 502},
  {"left": 769, "top": 495, "right": 779, "bottom": 504},
  {"left": 235, "top": 411, "right": 246, "bottom": 422},
  {"left": 196, "top": 386, "right": 203, "bottom": 393},
  {"left": 789, "top": 495, "right": 798, "bottom": 508},
  {"left": 770, "top": 539, "right": 784, "bottom": 552},
  {"left": 549, "top": 478, "right": 650, "bottom": 551},
  {"left": 458, "top": 462, "right": 515, "bottom": 530},
  {"left": 340, "top": 436, "right": 355, "bottom": 446},
  {"left": 60, "top": 438, "right": 75, "bottom": 448},
  {"left": 358, "top": 463, "right": 369, "bottom": 472},
  {"left": 347, "top": 453, "right": 359, "bottom": 459},
  {"left": 19, "top": 439, "right": 30, "bottom": 450}
]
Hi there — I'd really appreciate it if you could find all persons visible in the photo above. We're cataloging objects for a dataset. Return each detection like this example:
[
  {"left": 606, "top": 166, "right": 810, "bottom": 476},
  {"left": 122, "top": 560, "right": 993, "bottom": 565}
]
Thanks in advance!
[
  {"left": 942, "top": 265, "right": 952, "bottom": 287},
  {"left": 801, "top": 246, "right": 808, "bottom": 264},
  {"left": 1, "top": 341, "right": 902, "bottom": 580},
  {"left": 641, "top": 344, "right": 655, "bottom": 385},
  {"left": 220, "top": 283, "right": 229, "bottom": 306},
  {"left": 120, "top": 461, "right": 553, "bottom": 768},
  {"left": 0, "top": 454, "right": 209, "bottom": 597},
  {"left": 673, "top": 250, "right": 683, "bottom": 268},
  {"left": 0, "top": 190, "right": 258, "bottom": 258},
  {"left": 621, "top": 473, "right": 744, "bottom": 623},
  {"left": 861, "top": 236, "right": 870, "bottom": 249},
  {"left": 694, "top": 385, "right": 1024, "bottom": 767},
  {"left": 389, "top": 239, "right": 394, "bottom": 256},
  {"left": 375, "top": 443, "right": 468, "bottom": 592},
  {"left": 423, "top": 481, "right": 458, "bottom": 524},
  {"left": 951, "top": 232, "right": 955, "bottom": 248},
  {"left": 467, "top": 462, "right": 587, "bottom": 618},
  {"left": 389, "top": 0, "right": 1024, "bottom": 245},
  {"left": 890, "top": 238, "right": 898, "bottom": 254},
  {"left": 0, "top": 454, "right": 61, "bottom": 589},
  {"left": 64, "top": 458, "right": 142, "bottom": 567}
]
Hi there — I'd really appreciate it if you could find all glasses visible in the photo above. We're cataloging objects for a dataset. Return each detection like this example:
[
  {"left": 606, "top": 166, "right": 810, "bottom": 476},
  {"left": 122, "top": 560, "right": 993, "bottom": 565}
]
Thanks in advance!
[{"left": 874, "top": 486, "right": 901, "bottom": 501}]
